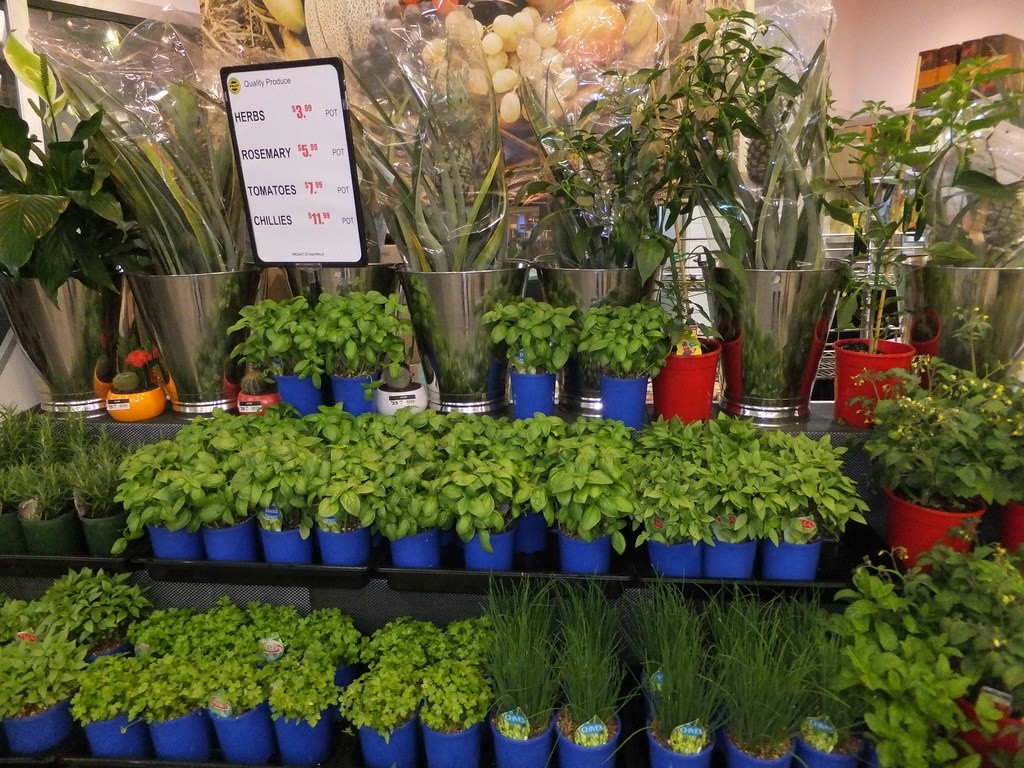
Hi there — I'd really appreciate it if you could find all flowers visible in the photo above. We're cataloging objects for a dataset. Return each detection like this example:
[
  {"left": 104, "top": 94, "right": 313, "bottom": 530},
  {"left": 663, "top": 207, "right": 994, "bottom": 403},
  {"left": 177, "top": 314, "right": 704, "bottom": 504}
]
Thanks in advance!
[{"left": 112, "top": 349, "right": 152, "bottom": 392}]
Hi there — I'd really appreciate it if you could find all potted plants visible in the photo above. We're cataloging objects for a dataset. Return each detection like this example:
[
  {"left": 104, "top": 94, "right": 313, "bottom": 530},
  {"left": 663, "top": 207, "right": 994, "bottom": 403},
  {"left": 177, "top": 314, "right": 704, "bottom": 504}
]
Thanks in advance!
[
  {"left": 0, "top": 401, "right": 21, "bottom": 552},
  {"left": 311, "top": 290, "right": 413, "bottom": 416},
  {"left": 74, "top": 423, "right": 129, "bottom": 556},
  {"left": 110, "top": 407, "right": 869, "bottom": 584},
  {"left": 343, "top": 17, "right": 524, "bottom": 417},
  {"left": 508, "top": 65, "right": 679, "bottom": 326},
  {"left": 0, "top": 29, "right": 118, "bottom": 413},
  {"left": 32, "top": 39, "right": 258, "bottom": 418},
  {"left": 1, "top": 567, "right": 1024, "bottom": 768},
  {"left": 821, "top": 56, "right": 1024, "bottom": 427},
  {"left": 652, "top": 309, "right": 722, "bottom": 426},
  {"left": 226, "top": 295, "right": 327, "bottom": 419},
  {"left": 678, "top": 7, "right": 849, "bottom": 424},
  {"left": 577, "top": 303, "right": 677, "bottom": 432},
  {"left": 17, "top": 414, "right": 75, "bottom": 555},
  {"left": 888, "top": 57, "right": 1024, "bottom": 383},
  {"left": 480, "top": 297, "right": 580, "bottom": 419},
  {"left": 848, "top": 307, "right": 1024, "bottom": 574},
  {"left": 207, "top": 0, "right": 401, "bottom": 299}
]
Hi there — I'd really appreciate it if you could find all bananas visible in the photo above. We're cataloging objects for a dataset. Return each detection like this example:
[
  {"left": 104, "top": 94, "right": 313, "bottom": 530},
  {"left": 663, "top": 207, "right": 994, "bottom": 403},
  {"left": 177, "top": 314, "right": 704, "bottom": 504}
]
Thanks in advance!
[
  {"left": 262, "top": 0, "right": 304, "bottom": 34},
  {"left": 623, "top": 1, "right": 660, "bottom": 75}
]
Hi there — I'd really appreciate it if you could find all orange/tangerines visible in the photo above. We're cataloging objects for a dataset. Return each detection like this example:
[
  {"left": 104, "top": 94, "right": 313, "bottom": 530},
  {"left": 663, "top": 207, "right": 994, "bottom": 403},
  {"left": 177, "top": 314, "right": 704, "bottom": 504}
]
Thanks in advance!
[{"left": 554, "top": 1, "right": 625, "bottom": 65}]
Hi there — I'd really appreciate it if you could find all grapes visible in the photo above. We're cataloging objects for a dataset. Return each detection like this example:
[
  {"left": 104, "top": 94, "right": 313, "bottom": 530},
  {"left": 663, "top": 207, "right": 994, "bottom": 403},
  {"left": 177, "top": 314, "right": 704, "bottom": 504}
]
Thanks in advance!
[{"left": 352, "top": 0, "right": 577, "bottom": 122}]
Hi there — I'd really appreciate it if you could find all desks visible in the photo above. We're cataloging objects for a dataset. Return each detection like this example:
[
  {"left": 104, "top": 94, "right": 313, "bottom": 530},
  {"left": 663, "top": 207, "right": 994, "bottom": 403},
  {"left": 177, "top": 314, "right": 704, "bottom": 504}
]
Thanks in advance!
[{"left": 16, "top": 403, "right": 655, "bottom": 447}]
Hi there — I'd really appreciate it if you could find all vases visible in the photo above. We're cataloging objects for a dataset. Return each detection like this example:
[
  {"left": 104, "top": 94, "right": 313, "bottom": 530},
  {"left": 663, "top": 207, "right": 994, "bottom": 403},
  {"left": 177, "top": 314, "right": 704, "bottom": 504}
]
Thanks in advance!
[{"left": 106, "top": 385, "right": 167, "bottom": 422}]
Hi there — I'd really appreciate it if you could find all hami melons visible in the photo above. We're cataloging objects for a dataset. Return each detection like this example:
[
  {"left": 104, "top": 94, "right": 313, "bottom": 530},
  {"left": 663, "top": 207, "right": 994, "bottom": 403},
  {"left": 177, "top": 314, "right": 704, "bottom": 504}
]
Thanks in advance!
[{"left": 305, "top": 0, "right": 400, "bottom": 105}]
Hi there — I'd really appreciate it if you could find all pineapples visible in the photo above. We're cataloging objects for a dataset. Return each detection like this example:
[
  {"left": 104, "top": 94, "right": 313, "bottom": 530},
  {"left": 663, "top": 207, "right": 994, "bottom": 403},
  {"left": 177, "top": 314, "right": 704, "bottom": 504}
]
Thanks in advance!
[
  {"left": 431, "top": 74, "right": 471, "bottom": 194},
  {"left": 747, "top": 100, "right": 781, "bottom": 183}
]
